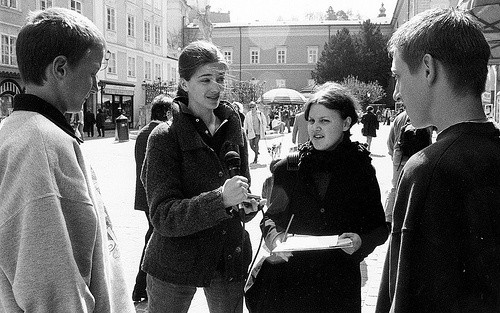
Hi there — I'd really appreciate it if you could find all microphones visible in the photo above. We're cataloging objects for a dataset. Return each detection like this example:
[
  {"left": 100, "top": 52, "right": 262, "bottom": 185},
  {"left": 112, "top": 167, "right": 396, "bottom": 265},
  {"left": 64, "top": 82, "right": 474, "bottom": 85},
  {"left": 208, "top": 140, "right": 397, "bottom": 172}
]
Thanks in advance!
[{"left": 224, "top": 151, "right": 245, "bottom": 219}]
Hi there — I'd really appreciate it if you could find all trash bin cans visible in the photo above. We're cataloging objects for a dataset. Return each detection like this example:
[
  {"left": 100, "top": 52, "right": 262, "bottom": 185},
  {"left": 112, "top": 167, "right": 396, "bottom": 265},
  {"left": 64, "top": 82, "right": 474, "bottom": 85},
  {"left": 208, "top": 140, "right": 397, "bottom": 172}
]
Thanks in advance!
[{"left": 116, "top": 114, "right": 130, "bottom": 143}]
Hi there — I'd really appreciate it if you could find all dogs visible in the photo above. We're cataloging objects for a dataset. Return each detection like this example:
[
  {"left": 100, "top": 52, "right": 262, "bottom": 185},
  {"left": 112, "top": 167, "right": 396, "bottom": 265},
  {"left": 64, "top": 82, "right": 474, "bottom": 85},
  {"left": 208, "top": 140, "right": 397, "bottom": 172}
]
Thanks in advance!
[{"left": 268, "top": 142, "right": 281, "bottom": 158}]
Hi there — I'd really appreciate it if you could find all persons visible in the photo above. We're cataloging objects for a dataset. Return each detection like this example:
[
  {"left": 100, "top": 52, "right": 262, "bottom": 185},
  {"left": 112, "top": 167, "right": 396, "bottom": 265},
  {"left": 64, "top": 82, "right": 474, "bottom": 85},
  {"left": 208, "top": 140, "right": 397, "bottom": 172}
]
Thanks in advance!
[
  {"left": 292, "top": 112, "right": 310, "bottom": 150},
  {"left": 394, "top": 122, "right": 433, "bottom": 165},
  {"left": 95, "top": 109, "right": 107, "bottom": 137},
  {"left": 361, "top": 107, "right": 379, "bottom": 151},
  {"left": 383, "top": 108, "right": 391, "bottom": 125},
  {"left": 272, "top": 116, "right": 286, "bottom": 134},
  {"left": 140, "top": 41, "right": 268, "bottom": 313},
  {"left": 232, "top": 102, "right": 246, "bottom": 127},
  {"left": 375, "top": 8, "right": 500, "bottom": 313},
  {"left": 384, "top": 111, "right": 414, "bottom": 221},
  {"left": 281, "top": 109, "right": 292, "bottom": 133},
  {"left": 132, "top": 94, "right": 181, "bottom": 301},
  {"left": 244, "top": 101, "right": 267, "bottom": 163},
  {"left": 259, "top": 80, "right": 389, "bottom": 313},
  {"left": 376, "top": 109, "right": 382, "bottom": 123},
  {"left": 83, "top": 108, "right": 95, "bottom": 137},
  {"left": 0, "top": 7, "right": 137, "bottom": 313}
]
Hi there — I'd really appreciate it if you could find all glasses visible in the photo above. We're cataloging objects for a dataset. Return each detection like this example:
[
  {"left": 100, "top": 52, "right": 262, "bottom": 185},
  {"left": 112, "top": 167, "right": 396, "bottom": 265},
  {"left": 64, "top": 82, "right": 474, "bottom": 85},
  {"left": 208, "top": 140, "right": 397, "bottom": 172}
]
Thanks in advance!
[{"left": 250, "top": 107, "right": 254, "bottom": 108}]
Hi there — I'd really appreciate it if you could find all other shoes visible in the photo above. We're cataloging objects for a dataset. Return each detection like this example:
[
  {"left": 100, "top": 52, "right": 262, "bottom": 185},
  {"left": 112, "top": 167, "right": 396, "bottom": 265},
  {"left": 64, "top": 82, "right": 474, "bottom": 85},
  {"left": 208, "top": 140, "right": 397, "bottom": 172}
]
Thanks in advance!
[{"left": 132, "top": 290, "right": 148, "bottom": 301}]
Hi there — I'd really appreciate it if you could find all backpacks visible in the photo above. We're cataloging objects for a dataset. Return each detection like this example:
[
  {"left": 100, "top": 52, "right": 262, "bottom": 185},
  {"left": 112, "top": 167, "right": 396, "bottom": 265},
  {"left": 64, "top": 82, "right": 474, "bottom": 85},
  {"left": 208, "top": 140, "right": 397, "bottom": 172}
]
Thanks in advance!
[{"left": 399, "top": 118, "right": 431, "bottom": 160}]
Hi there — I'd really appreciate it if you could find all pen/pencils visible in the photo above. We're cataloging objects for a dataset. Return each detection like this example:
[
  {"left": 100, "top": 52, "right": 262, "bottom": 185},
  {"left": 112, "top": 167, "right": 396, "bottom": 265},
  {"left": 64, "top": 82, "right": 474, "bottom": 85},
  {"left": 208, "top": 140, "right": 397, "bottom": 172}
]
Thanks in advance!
[{"left": 275, "top": 214, "right": 297, "bottom": 257}]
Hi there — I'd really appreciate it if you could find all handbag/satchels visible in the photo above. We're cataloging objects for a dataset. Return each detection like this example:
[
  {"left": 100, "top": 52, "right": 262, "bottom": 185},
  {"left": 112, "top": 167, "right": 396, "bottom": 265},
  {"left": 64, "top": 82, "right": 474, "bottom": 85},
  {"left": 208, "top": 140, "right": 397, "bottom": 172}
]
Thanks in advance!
[
  {"left": 361, "top": 128, "right": 367, "bottom": 136},
  {"left": 244, "top": 256, "right": 287, "bottom": 313},
  {"left": 242, "top": 228, "right": 253, "bottom": 274}
]
